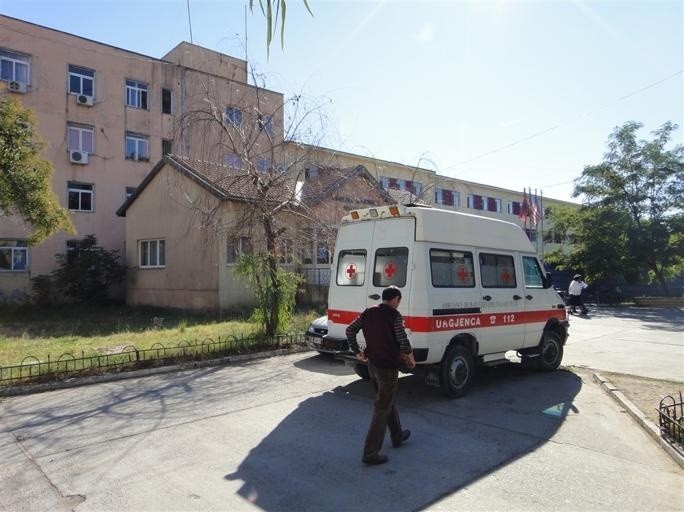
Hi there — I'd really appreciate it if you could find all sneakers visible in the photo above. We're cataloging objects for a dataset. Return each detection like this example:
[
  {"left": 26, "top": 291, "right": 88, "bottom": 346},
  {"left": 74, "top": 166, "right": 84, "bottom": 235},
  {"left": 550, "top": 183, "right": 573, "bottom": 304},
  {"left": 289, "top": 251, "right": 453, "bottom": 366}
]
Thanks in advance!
[
  {"left": 390, "top": 429, "right": 411, "bottom": 449},
  {"left": 361, "top": 454, "right": 389, "bottom": 466}
]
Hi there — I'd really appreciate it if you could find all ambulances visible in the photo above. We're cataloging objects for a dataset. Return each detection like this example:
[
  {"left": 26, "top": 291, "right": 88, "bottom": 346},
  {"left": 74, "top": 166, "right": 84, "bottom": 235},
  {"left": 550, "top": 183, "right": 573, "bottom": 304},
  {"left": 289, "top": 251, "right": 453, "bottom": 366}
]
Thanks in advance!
[{"left": 324, "top": 204, "right": 574, "bottom": 399}]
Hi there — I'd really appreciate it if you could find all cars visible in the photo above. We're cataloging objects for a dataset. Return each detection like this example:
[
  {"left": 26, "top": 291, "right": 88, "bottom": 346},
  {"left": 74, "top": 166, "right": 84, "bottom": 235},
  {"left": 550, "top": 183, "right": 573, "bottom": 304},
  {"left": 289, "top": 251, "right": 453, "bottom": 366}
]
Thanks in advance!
[{"left": 304, "top": 314, "right": 354, "bottom": 360}]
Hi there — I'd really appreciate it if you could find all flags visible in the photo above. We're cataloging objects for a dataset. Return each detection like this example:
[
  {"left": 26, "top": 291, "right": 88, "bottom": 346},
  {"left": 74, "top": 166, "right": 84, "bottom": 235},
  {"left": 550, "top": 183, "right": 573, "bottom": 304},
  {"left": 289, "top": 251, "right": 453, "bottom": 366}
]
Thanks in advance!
[
  {"left": 533, "top": 196, "right": 542, "bottom": 226},
  {"left": 528, "top": 193, "right": 540, "bottom": 228},
  {"left": 518, "top": 192, "right": 530, "bottom": 224}
]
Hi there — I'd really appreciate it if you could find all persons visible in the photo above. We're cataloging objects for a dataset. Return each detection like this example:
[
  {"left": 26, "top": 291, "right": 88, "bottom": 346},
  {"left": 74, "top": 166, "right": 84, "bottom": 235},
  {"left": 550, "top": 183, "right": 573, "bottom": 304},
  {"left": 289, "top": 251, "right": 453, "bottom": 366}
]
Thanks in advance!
[
  {"left": 572, "top": 276, "right": 591, "bottom": 313},
  {"left": 342, "top": 284, "right": 416, "bottom": 465},
  {"left": 566, "top": 272, "right": 588, "bottom": 315}
]
